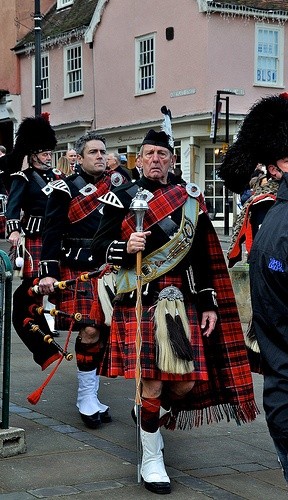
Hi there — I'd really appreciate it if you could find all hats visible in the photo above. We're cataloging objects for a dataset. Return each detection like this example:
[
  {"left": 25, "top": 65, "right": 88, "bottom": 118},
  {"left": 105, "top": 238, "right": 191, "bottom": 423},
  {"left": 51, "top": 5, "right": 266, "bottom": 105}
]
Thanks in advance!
[
  {"left": 239, "top": 92, "right": 288, "bottom": 164},
  {"left": 141, "top": 105, "right": 175, "bottom": 153},
  {"left": 216, "top": 141, "right": 259, "bottom": 194},
  {"left": 14, "top": 113, "right": 57, "bottom": 155}
]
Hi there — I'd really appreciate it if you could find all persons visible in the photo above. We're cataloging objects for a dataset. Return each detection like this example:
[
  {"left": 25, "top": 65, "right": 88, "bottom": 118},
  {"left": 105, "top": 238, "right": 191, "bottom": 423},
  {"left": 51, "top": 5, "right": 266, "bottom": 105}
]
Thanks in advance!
[
  {"left": 225, "top": 152, "right": 288, "bottom": 270},
  {"left": 36, "top": 131, "right": 129, "bottom": 428},
  {"left": 5, "top": 134, "right": 67, "bottom": 337},
  {"left": 247, "top": 170, "right": 288, "bottom": 488},
  {"left": 0, "top": 141, "right": 183, "bottom": 187},
  {"left": 91, "top": 128, "right": 220, "bottom": 494}
]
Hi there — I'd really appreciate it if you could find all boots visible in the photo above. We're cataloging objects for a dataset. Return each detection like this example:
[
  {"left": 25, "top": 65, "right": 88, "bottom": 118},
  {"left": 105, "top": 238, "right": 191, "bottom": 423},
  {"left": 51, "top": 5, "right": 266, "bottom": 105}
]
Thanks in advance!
[
  {"left": 77, "top": 369, "right": 101, "bottom": 429},
  {"left": 43, "top": 295, "right": 60, "bottom": 337},
  {"left": 138, "top": 426, "right": 172, "bottom": 494},
  {"left": 75, "top": 373, "right": 112, "bottom": 422},
  {"left": 132, "top": 400, "right": 172, "bottom": 459}
]
